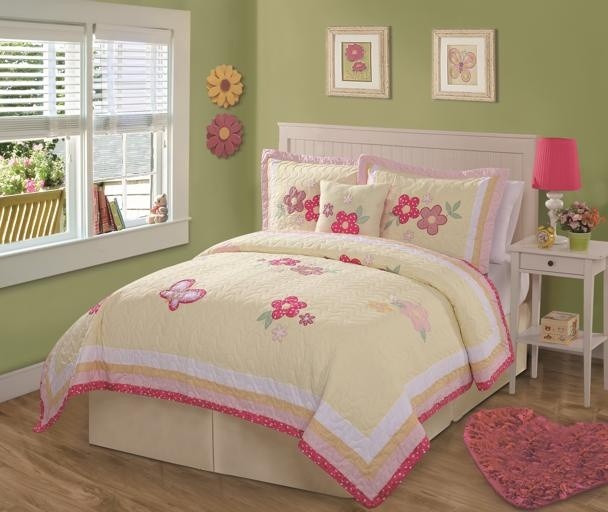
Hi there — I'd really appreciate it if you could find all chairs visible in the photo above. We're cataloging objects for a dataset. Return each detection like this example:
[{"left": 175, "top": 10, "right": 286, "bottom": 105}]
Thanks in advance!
[{"left": 0, "top": 186, "right": 67, "bottom": 245}]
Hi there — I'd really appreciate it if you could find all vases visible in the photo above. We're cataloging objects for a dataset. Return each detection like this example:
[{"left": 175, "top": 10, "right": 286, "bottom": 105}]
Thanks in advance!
[{"left": 569, "top": 230, "right": 591, "bottom": 252}]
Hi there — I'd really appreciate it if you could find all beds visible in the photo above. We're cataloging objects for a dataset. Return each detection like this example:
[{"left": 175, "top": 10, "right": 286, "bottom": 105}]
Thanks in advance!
[{"left": 32, "top": 121, "right": 543, "bottom": 508}]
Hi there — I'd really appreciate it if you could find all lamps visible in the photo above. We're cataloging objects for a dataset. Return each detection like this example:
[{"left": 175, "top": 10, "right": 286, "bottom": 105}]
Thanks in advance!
[{"left": 530, "top": 137, "right": 582, "bottom": 246}]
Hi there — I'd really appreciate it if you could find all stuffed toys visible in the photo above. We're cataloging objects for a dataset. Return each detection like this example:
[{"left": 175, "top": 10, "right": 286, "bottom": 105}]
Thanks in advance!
[{"left": 145, "top": 193, "right": 169, "bottom": 224}]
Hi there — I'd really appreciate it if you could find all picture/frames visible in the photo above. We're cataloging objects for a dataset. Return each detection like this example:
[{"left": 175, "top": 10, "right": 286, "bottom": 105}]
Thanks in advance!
[
  {"left": 430, "top": 27, "right": 498, "bottom": 104},
  {"left": 324, "top": 24, "right": 393, "bottom": 100}
]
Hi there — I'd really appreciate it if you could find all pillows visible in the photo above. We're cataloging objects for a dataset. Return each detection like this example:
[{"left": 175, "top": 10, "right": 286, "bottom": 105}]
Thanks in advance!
[
  {"left": 313, "top": 179, "right": 392, "bottom": 239},
  {"left": 257, "top": 145, "right": 366, "bottom": 234},
  {"left": 484, "top": 178, "right": 526, "bottom": 266},
  {"left": 355, "top": 152, "right": 512, "bottom": 279}
]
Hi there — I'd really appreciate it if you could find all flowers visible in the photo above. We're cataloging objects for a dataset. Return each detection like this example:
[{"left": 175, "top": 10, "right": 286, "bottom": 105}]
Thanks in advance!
[
  {"left": 557, "top": 199, "right": 606, "bottom": 235},
  {"left": 204, "top": 114, "right": 245, "bottom": 160},
  {"left": 345, "top": 44, "right": 368, "bottom": 79},
  {"left": 204, "top": 62, "right": 245, "bottom": 111}
]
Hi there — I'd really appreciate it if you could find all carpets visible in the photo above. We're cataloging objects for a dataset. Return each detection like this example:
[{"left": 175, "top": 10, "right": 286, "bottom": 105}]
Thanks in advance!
[{"left": 463, "top": 403, "right": 607, "bottom": 511}]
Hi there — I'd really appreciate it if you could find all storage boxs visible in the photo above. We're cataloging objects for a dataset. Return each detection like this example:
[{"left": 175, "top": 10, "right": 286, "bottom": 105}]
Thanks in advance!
[{"left": 540, "top": 308, "right": 580, "bottom": 345}]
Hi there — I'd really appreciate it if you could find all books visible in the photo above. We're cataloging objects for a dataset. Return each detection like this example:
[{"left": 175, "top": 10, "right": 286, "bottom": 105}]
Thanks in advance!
[{"left": 92, "top": 181, "right": 126, "bottom": 234}]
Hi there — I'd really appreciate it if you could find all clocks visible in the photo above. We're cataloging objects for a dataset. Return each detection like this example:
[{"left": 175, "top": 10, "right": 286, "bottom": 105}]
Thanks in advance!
[{"left": 535, "top": 223, "right": 555, "bottom": 250}]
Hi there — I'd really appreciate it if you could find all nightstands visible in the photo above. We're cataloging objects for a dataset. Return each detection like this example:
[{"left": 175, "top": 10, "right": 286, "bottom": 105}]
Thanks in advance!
[{"left": 503, "top": 230, "right": 608, "bottom": 409}]
